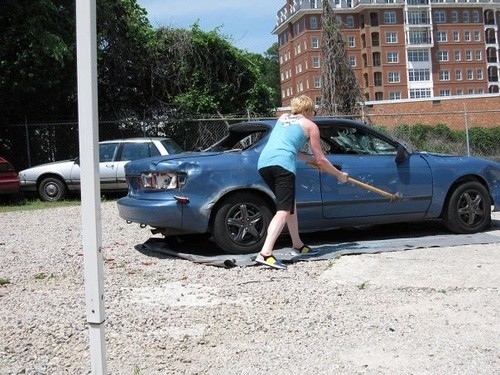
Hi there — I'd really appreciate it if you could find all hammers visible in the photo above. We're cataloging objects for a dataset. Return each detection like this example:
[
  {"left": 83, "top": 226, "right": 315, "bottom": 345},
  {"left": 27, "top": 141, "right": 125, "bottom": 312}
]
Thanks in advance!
[{"left": 307, "top": 161, "right": 402, "bottom": 202}]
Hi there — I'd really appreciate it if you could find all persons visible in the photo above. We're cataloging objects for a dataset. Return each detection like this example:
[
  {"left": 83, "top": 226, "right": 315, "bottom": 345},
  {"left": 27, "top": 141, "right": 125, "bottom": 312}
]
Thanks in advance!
[{"left": 255, "top": 95, "right": 348, "bottom": 270}]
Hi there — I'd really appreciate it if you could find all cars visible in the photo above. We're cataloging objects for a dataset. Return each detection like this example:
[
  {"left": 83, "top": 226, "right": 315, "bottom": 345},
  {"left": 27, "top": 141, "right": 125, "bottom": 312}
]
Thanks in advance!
[
  {"left": 0, "top": 157, "right": 20, "bottom": 193},
  {"left": 18, "top": 136, "right": 180, "bottom": 203},
  {"left": 116, "top": 119, "right": 500, "bottom": 256}
]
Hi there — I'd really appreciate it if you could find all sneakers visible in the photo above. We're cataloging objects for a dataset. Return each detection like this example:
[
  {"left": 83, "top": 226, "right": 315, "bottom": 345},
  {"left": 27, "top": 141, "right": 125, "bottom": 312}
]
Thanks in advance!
[
  {"left": 291, "top": 245, "right": 319, "bottom": 256},
  {"left": 255, "top": 253, "right": 287, "bottom": 269}
]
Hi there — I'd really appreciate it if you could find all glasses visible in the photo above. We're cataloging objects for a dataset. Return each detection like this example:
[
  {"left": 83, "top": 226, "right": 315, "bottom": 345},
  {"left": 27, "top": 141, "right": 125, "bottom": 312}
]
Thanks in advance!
[{"left": 313, "top": 110, "right": 316, "bottom": 116}]
